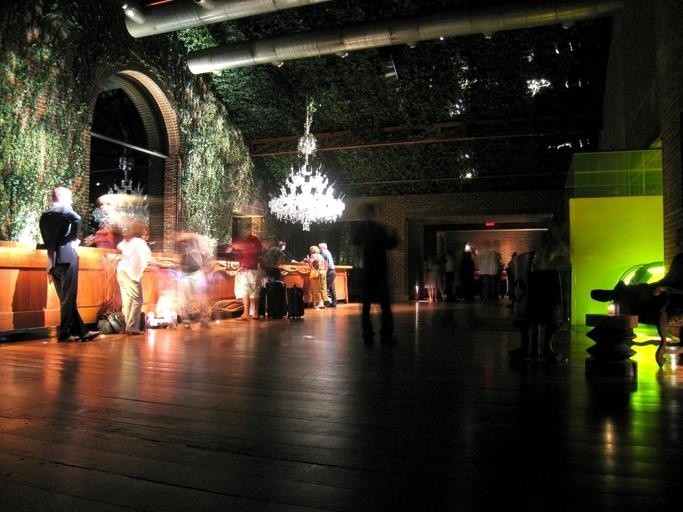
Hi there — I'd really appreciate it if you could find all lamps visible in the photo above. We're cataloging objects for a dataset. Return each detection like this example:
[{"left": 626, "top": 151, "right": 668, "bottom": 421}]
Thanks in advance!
[{"left": 267, "top": 98, "right": 347, "bottom": 232}]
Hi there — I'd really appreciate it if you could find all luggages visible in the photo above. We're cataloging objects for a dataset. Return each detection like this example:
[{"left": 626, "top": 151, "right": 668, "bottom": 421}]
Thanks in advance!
[{"left": 259, "top": 281, "right": 304, "bottom": 319}]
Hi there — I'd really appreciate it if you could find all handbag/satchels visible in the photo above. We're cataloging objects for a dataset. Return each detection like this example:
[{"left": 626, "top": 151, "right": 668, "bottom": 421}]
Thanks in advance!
[
  {"left": 97, "top": 313, "right": 145, "bottom": 334},
  {"left": 310, "top": 270, "right": 318, "bottom": 278}
]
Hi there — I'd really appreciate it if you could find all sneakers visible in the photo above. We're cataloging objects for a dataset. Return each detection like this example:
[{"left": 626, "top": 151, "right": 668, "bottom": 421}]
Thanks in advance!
[{"left": 60, "top": 330, "right": 100, "bottom": 341}]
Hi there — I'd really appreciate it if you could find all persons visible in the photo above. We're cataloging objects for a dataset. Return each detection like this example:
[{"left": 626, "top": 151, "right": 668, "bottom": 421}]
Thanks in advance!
[
  {"left": 258, "top": 235, "right": 335, "bottom": 318},
  {"left": 225, "top": 222, "right": 263, "bottom": 322},
  {"left": 115, "top": 220, "right": 173, "bottom": 335},
  {"left": 168, "top": 231, "right": 213, "bottom": 329},
  {"left": 419, "top": 215, "right": 571, "bottom": 357},
  {"left": 38, "top": 185, "right": 102, "bottom": 344},
  {"left": 589, "top": 224, "right": 683, "bottom": 326},
  {"left": 347, "top": 201, "right": 403, "bottom": 344}
]
